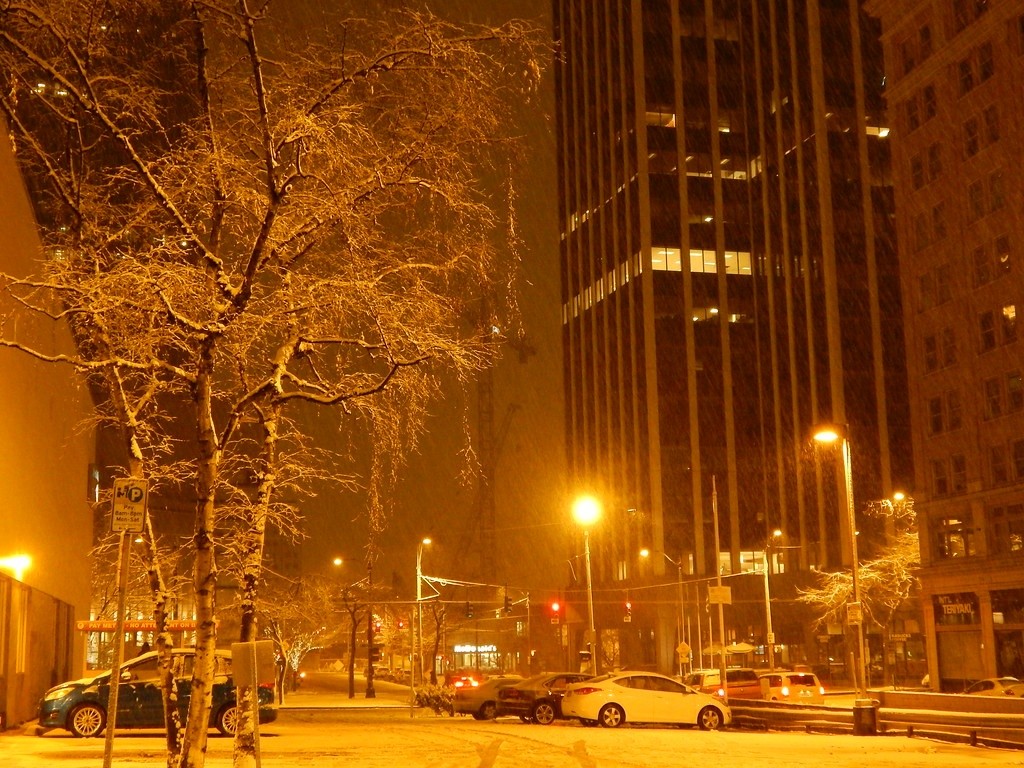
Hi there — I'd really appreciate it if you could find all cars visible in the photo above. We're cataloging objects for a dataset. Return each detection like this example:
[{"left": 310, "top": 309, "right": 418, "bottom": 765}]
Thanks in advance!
[
  {"left": 559, "top": 670, "right": 733, "bottom": 732},
  {"left": 496, "top": 673, "right": 597, "bottom": 725},
  {"left": 757, "top": 671, "right": 825, "bottom": 707},
  {"left": 959, "top": 676, "right": 1024, "bottom": 697},
  {"left": 684, "top": 668, "right": 762, "bottom": 700},
  {"left": 36, "top": 648, "right": 277, "bottom": 739},
  {"left": 451, "top": 677, "right": 529, "bottom": 720}
]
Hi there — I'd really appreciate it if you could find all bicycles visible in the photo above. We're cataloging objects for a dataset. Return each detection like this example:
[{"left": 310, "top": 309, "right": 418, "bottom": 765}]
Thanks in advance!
[{"left": 406, "top": 684, "right": 460, "bottom": 718}]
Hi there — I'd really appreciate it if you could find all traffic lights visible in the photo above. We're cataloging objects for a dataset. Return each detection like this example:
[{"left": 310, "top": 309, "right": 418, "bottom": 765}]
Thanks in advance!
[
  {"left": 505, "top": 595, "right": 513, "bottom": 613},
  {"left": 376, "top": 622, "right": 380, "bottom": 632},
  {"left": 467, "top": 600, "right": 474, "bottom": 618},
  {"left": 398, "top": 622, "right": 404, "bottom": 629},
  {"left": 552, "top": 600, "right": 560, "bottom": 619},
  {"left": 624, "top": 602, "right": 632, "bottom": 618}
]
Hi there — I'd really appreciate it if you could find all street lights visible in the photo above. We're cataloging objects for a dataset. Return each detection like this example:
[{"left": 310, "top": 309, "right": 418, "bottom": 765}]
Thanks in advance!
[
  {"left": 333, "top": 555, "right": 377, "bottom": 698},
  {"left": 573, "top": 499, "right": 603, "bottom": 679},
  {"left": 637, "top": 547, "right": 688, "bottom": 677},
  {"left": 813, "top": 423, "right": 867, "bottom": 696},
  {"left": 758, "top": 531, "right": 785, "bottom": 667},
  {"left": 416, "top": 536, "right": 432, "bottom": 683}
]
